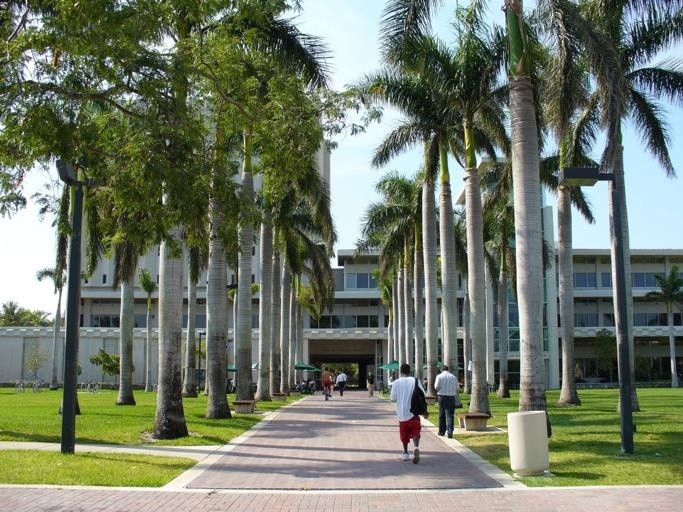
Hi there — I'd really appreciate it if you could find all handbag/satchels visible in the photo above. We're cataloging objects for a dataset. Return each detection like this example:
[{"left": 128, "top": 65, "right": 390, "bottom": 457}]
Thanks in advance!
[{"left": 410, "top": 387, "right": 428, "bottom": 416}]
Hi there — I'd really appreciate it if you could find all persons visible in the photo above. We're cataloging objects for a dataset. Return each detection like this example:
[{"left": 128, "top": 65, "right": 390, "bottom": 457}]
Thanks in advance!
[
  {"left": 365, "top": 369, "right": 375, "bottom": 396},
  {"left": 388, "top": 363, "right": 429, "bottom": 464},
  {"left": 422, "top": 377, "right": 428, "bottom": 391},
  {"left": 300, "top": 367, "right": 348, "bottom": 396},
  {"left": 433, "top": 364, "right": 461, "bottom": 438}
]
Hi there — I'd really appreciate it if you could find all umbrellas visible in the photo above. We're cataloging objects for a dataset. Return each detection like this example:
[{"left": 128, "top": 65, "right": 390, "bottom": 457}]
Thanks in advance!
[
  {"left": 225, "top": 360, "right": 321, "bottom": 373},
  {"left": 377, "top": 360, "right": 414, "bottom": 372},
  {"left": 422, "top": 360, "right": 442, "bottom": 369}
]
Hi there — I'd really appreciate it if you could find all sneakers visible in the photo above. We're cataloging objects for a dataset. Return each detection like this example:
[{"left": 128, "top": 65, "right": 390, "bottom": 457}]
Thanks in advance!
[
  {"left": 413, "top": 447, "right": 420, "bottom": 464},
  {"left": 438, "top": 432, "right": 452, "bottom": 438},
  {"left": 399, "top": 452, "right": 409, "bottom": 461}
]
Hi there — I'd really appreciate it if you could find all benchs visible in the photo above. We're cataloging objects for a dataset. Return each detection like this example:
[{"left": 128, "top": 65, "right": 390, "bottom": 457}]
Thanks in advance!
[
  {"left": 425, "top": 396, "right": 437, "bottom": 405},
  {"left": 232, "top": 399, "right": 259, "bottom": 414},
  {"left": 273, "top": 393, "right": 287, "bottom": 400},
  {"left": 458, "top": 413, "right": 490, "bottom": 431}
]
